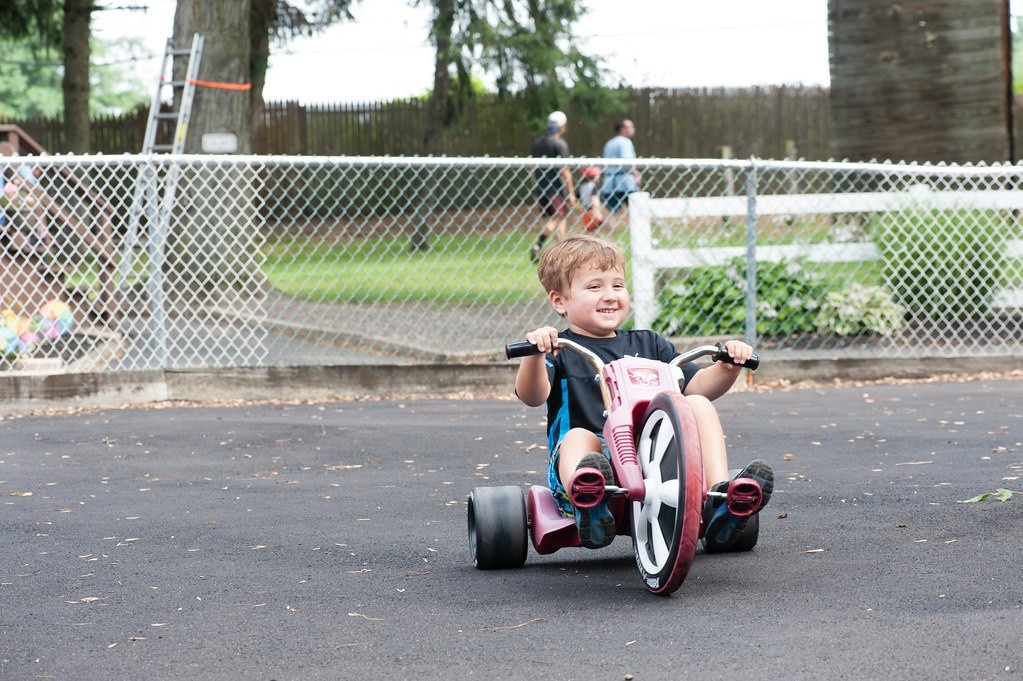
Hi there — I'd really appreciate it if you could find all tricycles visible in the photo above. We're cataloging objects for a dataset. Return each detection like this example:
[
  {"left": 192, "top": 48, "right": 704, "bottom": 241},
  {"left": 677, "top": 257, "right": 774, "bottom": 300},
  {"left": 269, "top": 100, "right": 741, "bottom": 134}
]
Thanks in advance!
[{"left": 466, "top": 337, "right": 761, "bottom": 595}]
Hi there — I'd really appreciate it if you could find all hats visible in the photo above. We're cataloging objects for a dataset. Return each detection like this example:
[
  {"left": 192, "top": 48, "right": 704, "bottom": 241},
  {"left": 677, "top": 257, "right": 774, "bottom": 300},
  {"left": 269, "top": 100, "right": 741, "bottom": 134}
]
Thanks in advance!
[
  {"left": 546, "top": 111, "right": 567, "bottom": 134},
  {"left": 579, "top": 165, "right": 600, "bottom": 179}
]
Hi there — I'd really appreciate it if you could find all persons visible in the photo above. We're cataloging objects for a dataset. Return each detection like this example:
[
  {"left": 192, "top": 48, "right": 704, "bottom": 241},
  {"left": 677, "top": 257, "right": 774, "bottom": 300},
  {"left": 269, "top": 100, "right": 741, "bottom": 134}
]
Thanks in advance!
[
  {"left": 602, "top": 118, "right": 641, "bottom": 212},
  {"left": 579, "top": 167, "right": 602, "bottom": 232},
  {"left": 531, "top": 111, "right": 577, "bottom": 264},
  {"left": 515, "top": 234, "right": 776, "bottom": 550}
]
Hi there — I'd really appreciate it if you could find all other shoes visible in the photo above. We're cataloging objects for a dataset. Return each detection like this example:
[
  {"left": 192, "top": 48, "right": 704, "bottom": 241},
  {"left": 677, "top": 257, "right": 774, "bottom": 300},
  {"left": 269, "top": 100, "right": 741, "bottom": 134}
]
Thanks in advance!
[
  {"left": 571, "top": 451, "right": 616, "bottom": 549},
  {"left": 530, "top": 245, "right": 540, "bottom": 263},
  {"left": 701, "top": 458, "right": 774, "bottom": 554}
]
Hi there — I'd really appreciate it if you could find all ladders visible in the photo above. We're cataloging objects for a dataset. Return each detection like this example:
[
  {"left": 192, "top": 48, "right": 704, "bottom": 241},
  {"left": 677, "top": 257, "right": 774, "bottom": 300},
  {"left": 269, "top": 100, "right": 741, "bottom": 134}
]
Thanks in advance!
[{"left": 112, "top": 32, "right": 205, "bottom": 366}]
